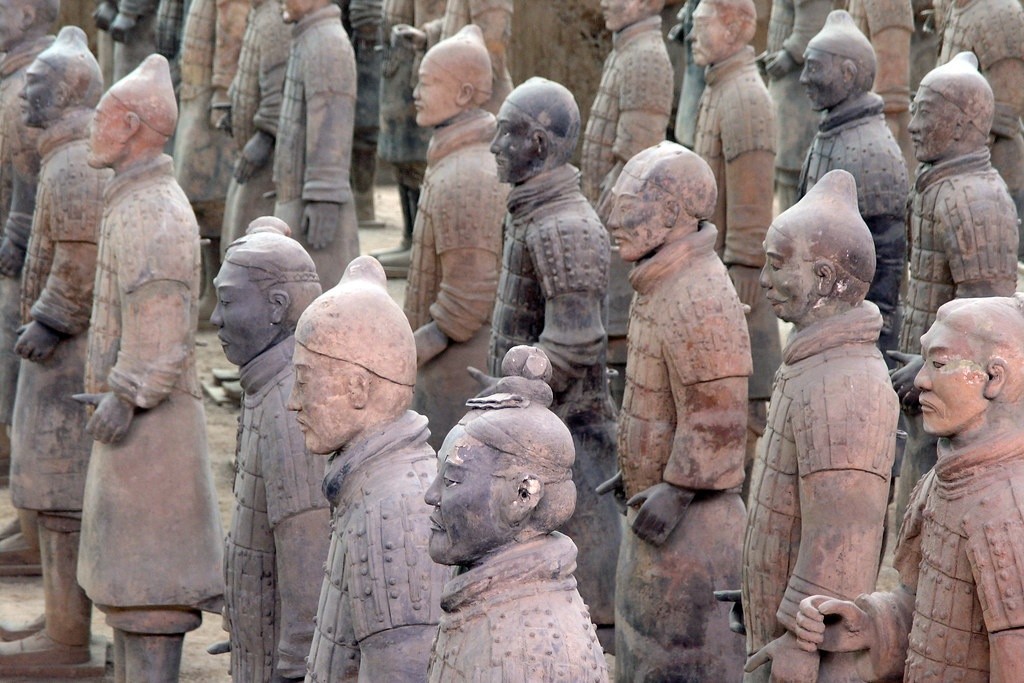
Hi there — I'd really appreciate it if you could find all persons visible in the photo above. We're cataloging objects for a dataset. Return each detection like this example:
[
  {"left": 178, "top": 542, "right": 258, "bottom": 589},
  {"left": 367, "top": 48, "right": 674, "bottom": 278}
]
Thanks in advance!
[
  {"left": 261, "top": 0, "right": 357, "bottom": 296},
  {"left": 579, "top": 0, "right": 675, "bottom": 360},
  {"left": 0, "top": 25, "right": 114, "bottom": 665},
  {"left": 215, "top": 0, "right": 295, "bottom": 257},
  {"left": 595, "top": 139, "right": 756, "bottom": 683},
  {"left": 687, "top": 0, "right": 782, "bottom": 400},
  {"left": 286, "top": 254, "right": 458, "bottom": 682},
  {"left": 463, "top": 74, "right": 622, "bottom": 651},
  {"left": 209, "top": 215, "right": 330, "bottom": 683},
  {"left": 713, "top": 168, "right": 899, "bottom": 682},
  {"left": 333, "top": 0, "right": 515, "bottom": 264},
  {"left": 170, "top": 0, "right": 254, "bottom": 323},
  {"left": 0, "top": 0, "right": 58, "bottom": 475},
  {"left": 668, "top": 0, "right": 1023, "bottom": 541},
  {"left": 424, "top": 346, "right": 611, "bottom": 683},
  {"left": 887, "top": 50, "right": 1020, "bottom": 536},
  {"left": 402, "top": 25, "right": 513, "bottom": 444},
  {"left": 92, "top": 0, "right": 190, "bottom": 158},
  {"left": 794, "top": 298, "right": 1023, "bottom": 682},
  {"left": 70, "top": 52, "right": 226, "bottom": 683}
]
